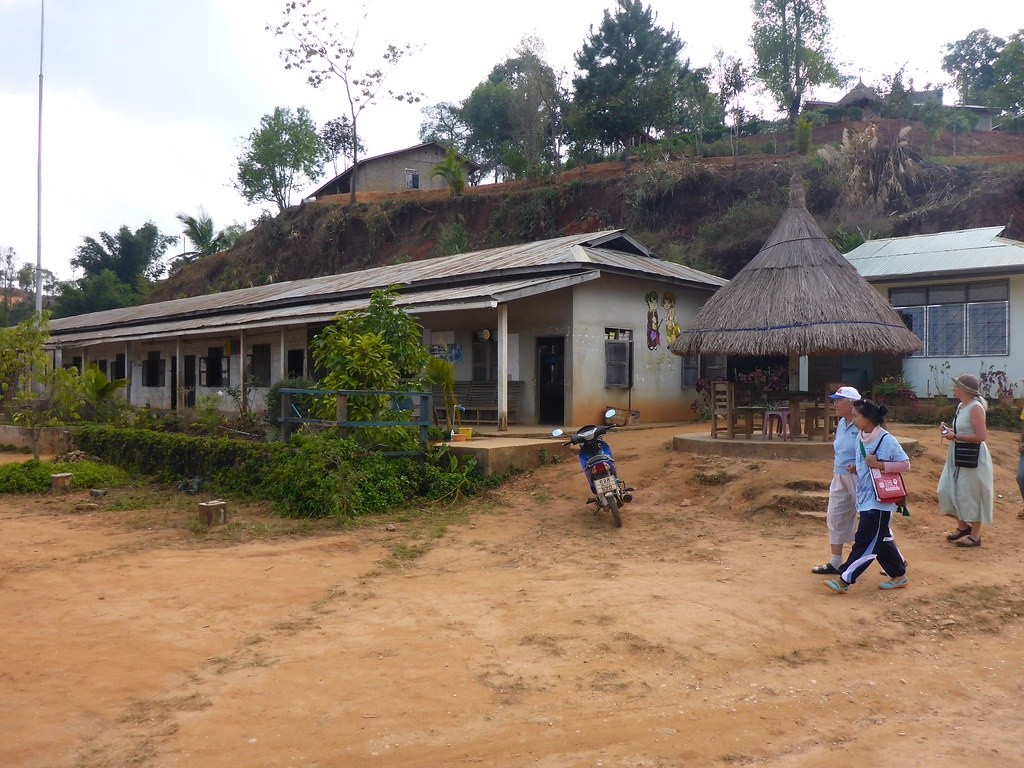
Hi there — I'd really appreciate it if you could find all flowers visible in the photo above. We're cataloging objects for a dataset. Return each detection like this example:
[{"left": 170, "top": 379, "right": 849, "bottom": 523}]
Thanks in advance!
[
  {"left": 995, "top": 371, "right": 1018, "bottom": 396},
  {"left": 979, "top": 362, "right": 996, "bottom": 390}
]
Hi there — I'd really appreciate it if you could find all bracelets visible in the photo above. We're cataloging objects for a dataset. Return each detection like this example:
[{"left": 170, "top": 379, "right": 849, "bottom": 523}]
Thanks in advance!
[{"left": 953, "top": 434, "right": 956, "bottom": 440}]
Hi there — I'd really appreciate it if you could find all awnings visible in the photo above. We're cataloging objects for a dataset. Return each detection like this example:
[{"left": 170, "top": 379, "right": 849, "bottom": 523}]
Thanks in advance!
[{"left": 41, "top": 268, "right": 597, "bottom": 349}]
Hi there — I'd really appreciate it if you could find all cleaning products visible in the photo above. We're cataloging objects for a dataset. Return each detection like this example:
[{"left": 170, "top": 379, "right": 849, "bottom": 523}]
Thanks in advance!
[{"left": 450, "top": 430, "right": 454, "bottom": 441}]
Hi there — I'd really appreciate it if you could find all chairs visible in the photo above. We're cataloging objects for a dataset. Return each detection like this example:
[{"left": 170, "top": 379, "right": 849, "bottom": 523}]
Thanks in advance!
[{"left": 710, "top": 381, "right": 850, "bottom": 442}]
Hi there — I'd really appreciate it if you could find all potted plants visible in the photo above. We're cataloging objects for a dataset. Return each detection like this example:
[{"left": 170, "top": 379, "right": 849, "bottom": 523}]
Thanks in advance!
[
  {"left": 929, "top": 360, "right": 950, "bottom": 406},
  {"left": 896, "top": 377, "right": 916, "bottom": 399}
]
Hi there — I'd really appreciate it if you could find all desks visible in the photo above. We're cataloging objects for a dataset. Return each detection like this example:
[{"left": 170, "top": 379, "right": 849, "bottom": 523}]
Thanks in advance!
[{"left": 760, "top": 390, "right": 821, "bottom": 440}]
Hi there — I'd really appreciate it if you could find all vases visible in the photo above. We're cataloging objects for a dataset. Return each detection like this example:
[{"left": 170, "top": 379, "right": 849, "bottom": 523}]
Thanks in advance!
[
  {"left": 983, "top": 390, "right": 990, "bottom": 398},
  {"left": 998, "top": 395, "right": 1014, "bottom": 408}
]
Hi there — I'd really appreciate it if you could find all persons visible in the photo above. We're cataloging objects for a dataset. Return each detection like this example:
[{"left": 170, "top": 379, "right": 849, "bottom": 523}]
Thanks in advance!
[
  {"left": 812, "top": 386, "right": 908, "bottom": 576},
  {"left": 822, "top": 399, "right": 911, "bottom": 595},
  {"left": 1015, "top": 407, "right": 1024, "bottom": 520},
  {"left": 936, "top": 374, "right": 994, "bottom": 547}
]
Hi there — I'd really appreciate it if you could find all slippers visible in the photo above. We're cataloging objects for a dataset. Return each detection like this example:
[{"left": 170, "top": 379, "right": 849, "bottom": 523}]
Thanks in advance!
[{"left": 812, "top": 562, "right": 839, "bottom": 574}]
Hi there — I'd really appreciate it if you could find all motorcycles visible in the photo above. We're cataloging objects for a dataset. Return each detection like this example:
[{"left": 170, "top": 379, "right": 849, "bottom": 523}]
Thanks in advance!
[{"left": 552, "top": 409, "right": 635, "bottom": 528}]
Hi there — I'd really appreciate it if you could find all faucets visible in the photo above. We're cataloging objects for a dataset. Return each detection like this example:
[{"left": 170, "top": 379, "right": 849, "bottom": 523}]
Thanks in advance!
[{"left": 455, "top": 404, "right": 465, "bottom": 412}]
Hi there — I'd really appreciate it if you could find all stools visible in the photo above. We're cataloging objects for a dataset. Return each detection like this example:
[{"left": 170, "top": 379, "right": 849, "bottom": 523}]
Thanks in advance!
[{"left": 762, "top": 411, "right": 794, "bottom": 442}]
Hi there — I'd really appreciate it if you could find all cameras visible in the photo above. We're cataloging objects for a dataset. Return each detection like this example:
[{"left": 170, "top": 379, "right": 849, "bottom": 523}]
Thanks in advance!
[{"left": 941, "top": 425, "right": 948, "bottom": 434}]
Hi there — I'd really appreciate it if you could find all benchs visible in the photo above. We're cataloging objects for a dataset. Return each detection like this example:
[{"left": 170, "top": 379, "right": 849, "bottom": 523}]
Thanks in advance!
[{"left": 431, "top": 380, "right": 524, "bottom": 426}]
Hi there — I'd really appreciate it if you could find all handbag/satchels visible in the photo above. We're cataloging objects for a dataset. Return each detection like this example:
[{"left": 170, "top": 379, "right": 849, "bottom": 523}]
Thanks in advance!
[
  {"left": 867, "top": 433, "right": 907, "bottom": 503},
  {"left": 954, "top": 441, "right": 981, "bottom": 468}
]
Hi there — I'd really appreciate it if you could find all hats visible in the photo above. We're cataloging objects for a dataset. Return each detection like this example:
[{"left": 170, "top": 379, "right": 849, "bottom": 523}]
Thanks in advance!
[
  {"left": 951, "top": 374, "right": 982, "bottom": 395},
  {"left": 828, "top": 386, "right": 862, "bottom": 400}
]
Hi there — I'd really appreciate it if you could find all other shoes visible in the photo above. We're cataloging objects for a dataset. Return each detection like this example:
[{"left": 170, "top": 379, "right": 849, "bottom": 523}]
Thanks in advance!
[
  {"left": 879, "top": 576, "right": 908, "bottom": 589},
  {"left": 955, "top": 535, "right": 982, "bottom": 547},
  {"left": 1018, "top": 509, "right": 1024, "bottom": 519},
  {"left": 946, "top": 524, "right": 972, "bottom": 540},
  {"left": 880, "top": 570, "right": 887, "bottom": 576},
  {"left": 822, "top": 579, "right": 848, "bottom": 594}
]
